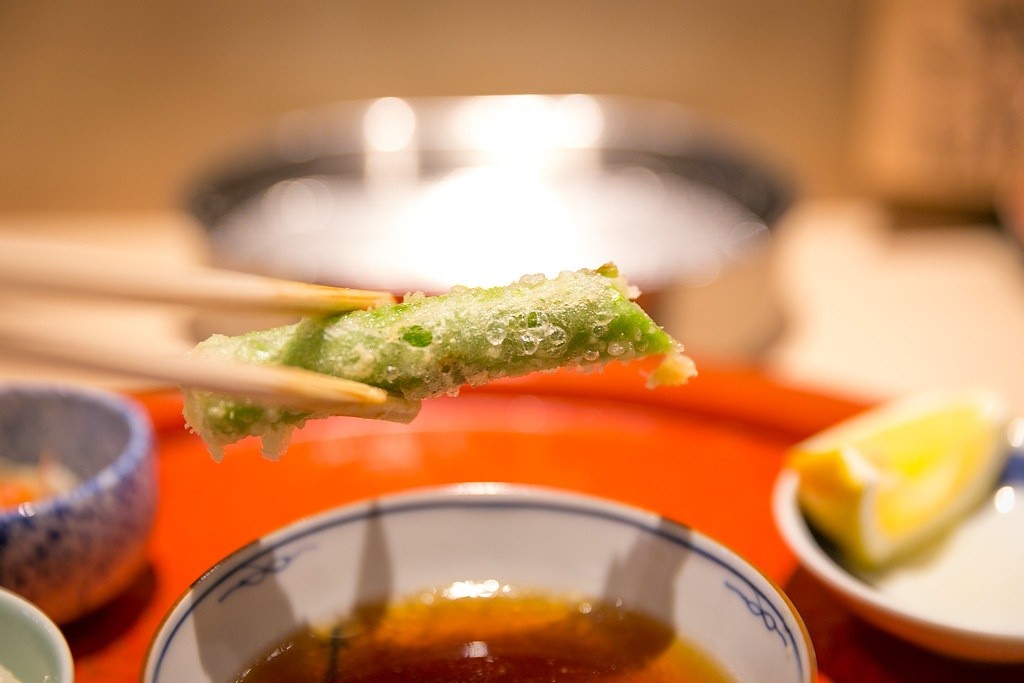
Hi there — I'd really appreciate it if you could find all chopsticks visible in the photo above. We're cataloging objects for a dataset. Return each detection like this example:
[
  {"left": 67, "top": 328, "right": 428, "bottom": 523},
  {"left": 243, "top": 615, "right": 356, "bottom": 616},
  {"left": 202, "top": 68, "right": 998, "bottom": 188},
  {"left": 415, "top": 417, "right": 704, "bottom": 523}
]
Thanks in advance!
[{"left": 0, "top": 239, "right": 422, "bottom": 424}]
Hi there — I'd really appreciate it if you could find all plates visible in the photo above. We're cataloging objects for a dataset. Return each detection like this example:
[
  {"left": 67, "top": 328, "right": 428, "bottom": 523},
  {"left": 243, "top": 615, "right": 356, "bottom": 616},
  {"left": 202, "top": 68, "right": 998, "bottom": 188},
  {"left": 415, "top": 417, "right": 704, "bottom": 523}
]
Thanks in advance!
[{"left": 776, "top": 452, "right": 1024, "bottom": 661}]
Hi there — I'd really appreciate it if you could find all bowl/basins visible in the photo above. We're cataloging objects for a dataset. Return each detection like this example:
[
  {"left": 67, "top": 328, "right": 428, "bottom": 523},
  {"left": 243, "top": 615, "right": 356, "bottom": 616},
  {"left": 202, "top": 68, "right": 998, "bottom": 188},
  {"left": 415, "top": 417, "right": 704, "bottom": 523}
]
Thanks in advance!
[{"left": 143, "top": 486, "right": 817, "bottom": 683}]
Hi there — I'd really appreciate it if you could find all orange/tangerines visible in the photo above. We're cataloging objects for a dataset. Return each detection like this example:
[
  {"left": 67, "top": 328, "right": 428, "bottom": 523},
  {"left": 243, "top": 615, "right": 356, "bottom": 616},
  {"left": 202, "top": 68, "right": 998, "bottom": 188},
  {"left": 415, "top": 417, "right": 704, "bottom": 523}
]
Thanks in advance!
[{"left": 787, "top": 385, "right": 1011, "bottom": 568}]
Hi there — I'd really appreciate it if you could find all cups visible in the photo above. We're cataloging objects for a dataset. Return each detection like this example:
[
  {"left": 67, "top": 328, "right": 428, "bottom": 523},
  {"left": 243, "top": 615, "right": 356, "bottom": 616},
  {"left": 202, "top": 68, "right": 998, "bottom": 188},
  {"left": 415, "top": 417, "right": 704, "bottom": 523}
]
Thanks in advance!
[{"left": 0, "top": 385, "right": 152, "bottom": 627}]
